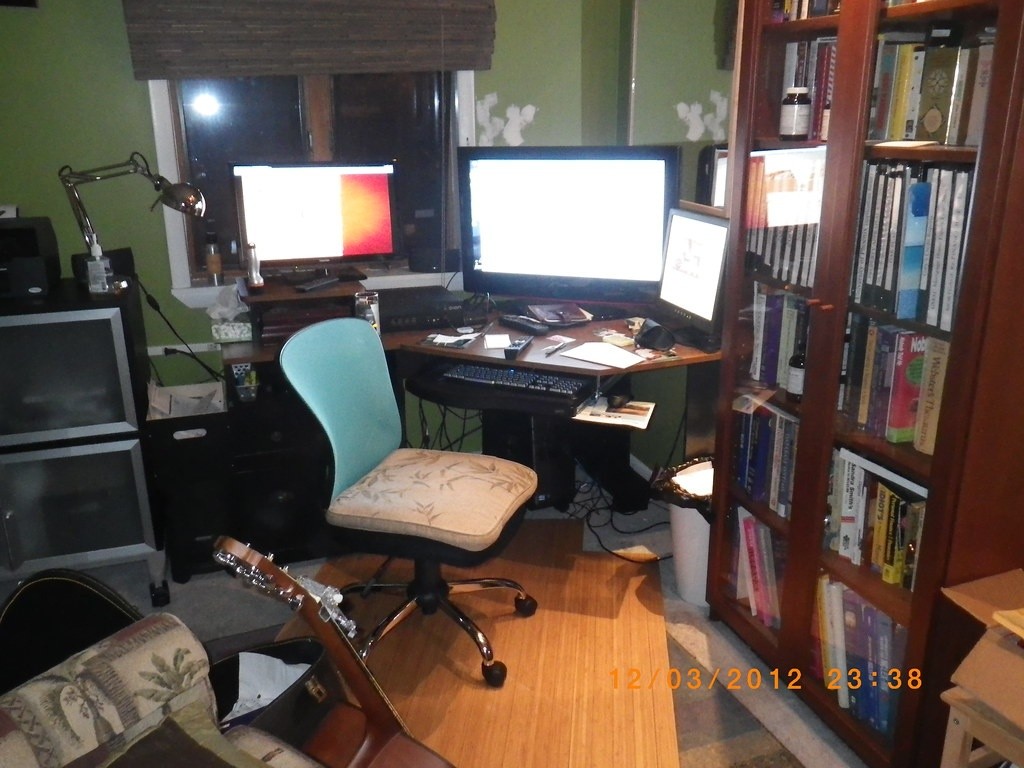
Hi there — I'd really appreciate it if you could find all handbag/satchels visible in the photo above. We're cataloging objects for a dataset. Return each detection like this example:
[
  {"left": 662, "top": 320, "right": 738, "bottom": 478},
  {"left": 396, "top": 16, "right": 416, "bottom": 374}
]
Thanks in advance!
[{"left": 209, "top": 634, "right": 346, "bottom": 746}]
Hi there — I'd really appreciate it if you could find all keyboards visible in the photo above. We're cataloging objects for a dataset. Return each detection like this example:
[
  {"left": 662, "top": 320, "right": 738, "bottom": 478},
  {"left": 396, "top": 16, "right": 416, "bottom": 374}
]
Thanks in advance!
[{"left": 443, "top": 364, "right": 584, "bottom": 395}]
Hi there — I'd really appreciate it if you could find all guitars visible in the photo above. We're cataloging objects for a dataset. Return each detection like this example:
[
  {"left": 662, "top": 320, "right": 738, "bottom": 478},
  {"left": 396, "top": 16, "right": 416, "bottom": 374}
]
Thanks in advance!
[{"left": 206, "top": 532, "right": 458, "bottom": 768}]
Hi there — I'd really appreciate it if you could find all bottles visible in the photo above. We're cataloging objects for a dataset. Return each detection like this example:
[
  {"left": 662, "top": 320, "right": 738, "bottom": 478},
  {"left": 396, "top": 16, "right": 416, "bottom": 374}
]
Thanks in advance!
[
  {"left": 779, "top": 87, "right": 812, "bottom": 142},
  {"left": 820, "top": 97, "right": 831, "bottom": 142},
  {"left": 247, "top": 242, "right": 264, "bottom": 291},
  {"left": 204, "top": 231, "right": 225, "bottom": 286},
  {"left": 786, "top": 344, "right": 806, "bottom": 403}
]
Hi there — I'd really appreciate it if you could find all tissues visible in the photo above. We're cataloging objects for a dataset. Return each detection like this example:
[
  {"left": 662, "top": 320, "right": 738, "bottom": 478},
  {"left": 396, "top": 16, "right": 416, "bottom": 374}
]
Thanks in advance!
[{"left": 204, "top": 285, "right": 253, "bottom": 344}]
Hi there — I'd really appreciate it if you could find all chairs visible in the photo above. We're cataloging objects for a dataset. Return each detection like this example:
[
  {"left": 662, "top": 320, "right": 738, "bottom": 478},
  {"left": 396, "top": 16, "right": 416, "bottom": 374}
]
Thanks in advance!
[{"left": 277, "top": 316, "right": 539, "bottom": 694}]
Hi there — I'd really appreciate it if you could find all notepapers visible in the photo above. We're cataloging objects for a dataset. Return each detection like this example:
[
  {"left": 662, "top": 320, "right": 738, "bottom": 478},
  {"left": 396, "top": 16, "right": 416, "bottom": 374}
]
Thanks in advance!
[{"left": 603, "top": 334, "right": 634, "bottom": 347}]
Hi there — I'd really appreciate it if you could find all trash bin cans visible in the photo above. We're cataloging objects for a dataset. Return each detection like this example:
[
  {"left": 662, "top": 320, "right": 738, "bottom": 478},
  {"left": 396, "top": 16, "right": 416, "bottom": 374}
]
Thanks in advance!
[{"left": 668, "top": 460, "right": 714, "bottom": 607}]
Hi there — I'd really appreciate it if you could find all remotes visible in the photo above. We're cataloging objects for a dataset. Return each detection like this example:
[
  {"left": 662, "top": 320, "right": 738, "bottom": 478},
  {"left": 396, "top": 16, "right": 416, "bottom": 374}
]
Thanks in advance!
[
  {"left": 505, "top": 335, "right": 535, "bottom": 360},
  {"left": 498, "top": 314, "right": 550, "bottom": 335},
  {"left": 295, "top": 277, "right": 339, "bottom": 291}
]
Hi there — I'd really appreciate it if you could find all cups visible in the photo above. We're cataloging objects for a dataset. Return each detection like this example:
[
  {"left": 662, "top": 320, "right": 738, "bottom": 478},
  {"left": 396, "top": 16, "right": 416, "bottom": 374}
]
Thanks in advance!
[
  {"left": 236, "top": 383, "right": 260, "bottom": 403},
  {"left": 355, "top": 292, "right": 381, "bottom": 340}
]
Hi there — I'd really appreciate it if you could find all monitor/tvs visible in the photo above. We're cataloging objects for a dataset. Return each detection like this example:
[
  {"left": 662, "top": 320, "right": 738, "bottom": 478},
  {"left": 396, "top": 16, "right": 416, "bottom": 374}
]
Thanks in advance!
[
  {"left": 457, "top": 144, "right": 682, "bottom": 321},
  {"left": 226, "top": 161, "right": 405, "bottom": 282},
  {"left": 656, "top": 208, "right": 730, "bottom": 350}
]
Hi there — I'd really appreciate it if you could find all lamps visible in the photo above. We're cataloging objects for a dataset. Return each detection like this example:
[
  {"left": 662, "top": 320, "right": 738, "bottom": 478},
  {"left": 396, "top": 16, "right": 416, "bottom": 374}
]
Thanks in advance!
[{"left": 58, "top": 148, "right": 207, "bottom": 293}]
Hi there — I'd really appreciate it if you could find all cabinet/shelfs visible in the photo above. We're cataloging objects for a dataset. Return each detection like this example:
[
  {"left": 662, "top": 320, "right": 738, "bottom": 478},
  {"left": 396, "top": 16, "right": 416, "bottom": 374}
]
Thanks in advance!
[
  {"left": 705, "top": 0, "right": 1024, "bottom": 768},
  {"left": 0, "top": 277, "right": 172, "bottom": 618},
  {"left": 221, "top": 268, "right": 725, "bottom": 547}
]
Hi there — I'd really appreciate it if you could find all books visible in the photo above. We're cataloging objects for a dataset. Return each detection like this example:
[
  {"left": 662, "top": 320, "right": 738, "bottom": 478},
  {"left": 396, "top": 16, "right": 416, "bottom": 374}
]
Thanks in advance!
[
  {"left": 849, "top": 160, "right": 975, "bottom": 335},
  {"left": 732, "top": 391, "right": 800, "bottom": 523},
  {"left": 782, "top": 36, "right": 837, "bottom": 143},
  {"left": 815, "top": 572, "right": 908, "bottom": 741},
  {"left": 771, "top": 0, "right": 840, "bottom": 23},
  {"left": 749, "top": 281, "right": 810, "bottom": 391},
  {"left": 528, "top": 302, "right": 587, "bottom": 322},
  {"left": 867, "top": 21, "right": 996, "bottom": 147},
  {"left": 745, "top": 150, "right": 826, "bottom": 290},
  {"left": 837, "top": 312, "right": 951, "bottom": 456},
  {"left": 821, "top": 445, "right": 928, "bottom": 594},
  {"left": 723, "top": 499, "right": 782, "bottom": 631},
  {"left": 885, "top": 0, "right": 931, "bottom": 7}
]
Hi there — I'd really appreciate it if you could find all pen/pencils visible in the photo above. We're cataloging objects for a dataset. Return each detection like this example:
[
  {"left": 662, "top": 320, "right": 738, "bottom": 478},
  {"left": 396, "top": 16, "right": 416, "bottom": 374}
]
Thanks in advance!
[
  {"left": 547, "top": 342, "right": 565, "bottom": 356},
  {"left": 481, "top": 322, "right": 493, "bottom": 337}
]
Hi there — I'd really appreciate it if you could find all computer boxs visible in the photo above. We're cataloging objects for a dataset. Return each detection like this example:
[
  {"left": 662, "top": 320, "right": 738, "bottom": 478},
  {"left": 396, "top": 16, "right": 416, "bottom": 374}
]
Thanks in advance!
[{"left": 481, "top": 408, "right": 576, "bottom": 512}]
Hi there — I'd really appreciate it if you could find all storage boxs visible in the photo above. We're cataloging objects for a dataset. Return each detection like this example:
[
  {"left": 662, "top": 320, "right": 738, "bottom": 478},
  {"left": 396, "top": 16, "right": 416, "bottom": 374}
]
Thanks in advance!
[{"left": 146, "top": 378, "right": 233, "bottom": 482}]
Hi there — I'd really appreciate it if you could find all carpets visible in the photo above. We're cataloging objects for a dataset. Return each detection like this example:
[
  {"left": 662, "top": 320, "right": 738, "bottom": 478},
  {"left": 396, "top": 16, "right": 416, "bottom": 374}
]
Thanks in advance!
[{"left": 201, "top": 623, "right": 805, "bottom": 768}]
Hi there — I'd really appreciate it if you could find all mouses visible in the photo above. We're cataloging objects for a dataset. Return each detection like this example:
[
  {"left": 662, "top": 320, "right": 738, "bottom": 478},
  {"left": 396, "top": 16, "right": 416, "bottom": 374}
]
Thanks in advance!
[{"left": 608, "top": 393, "right": 631, "bottom": 408}]
